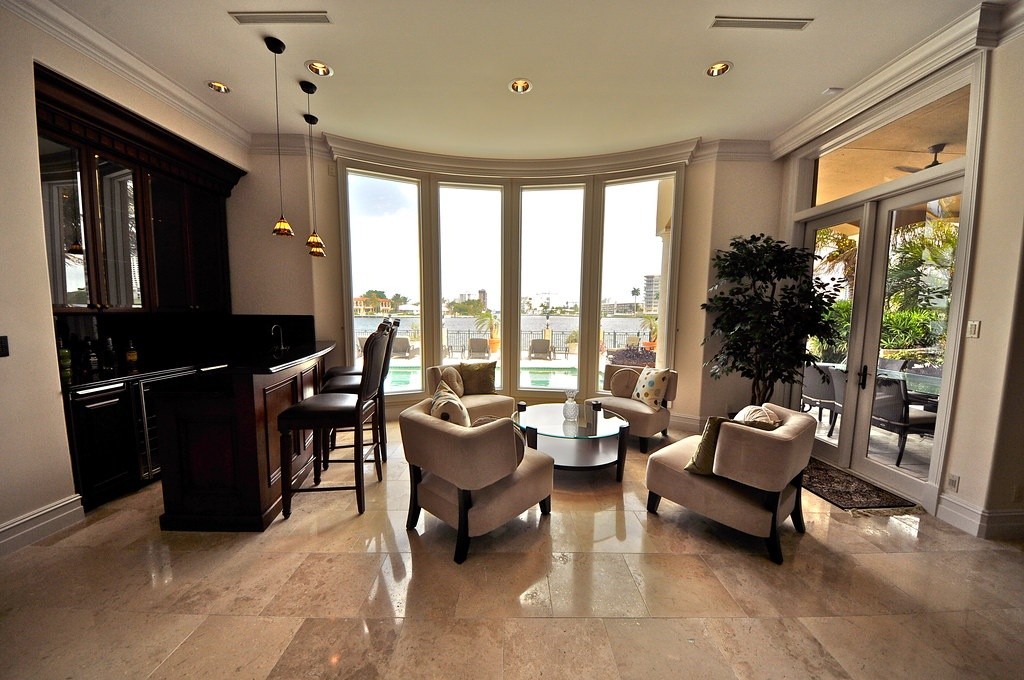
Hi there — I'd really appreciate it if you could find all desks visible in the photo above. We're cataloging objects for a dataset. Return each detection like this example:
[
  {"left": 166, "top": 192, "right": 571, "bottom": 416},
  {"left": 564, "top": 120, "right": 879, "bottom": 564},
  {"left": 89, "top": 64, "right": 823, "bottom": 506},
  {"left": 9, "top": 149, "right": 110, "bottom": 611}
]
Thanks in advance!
[
  {"left": 551, "top": 346, "right": 569, "bottom": 360},
  {"left": 606, "top": 348, "right": 619, "bottom": 359},
  {"left": 146, "top": 341, "right": 336, "bottom": 531},
  {"left": 876, "top": 369, "right": 942, "bottom": 439},
  {"left": 448, "top": 345, "right": 467, "bottom": 359}
]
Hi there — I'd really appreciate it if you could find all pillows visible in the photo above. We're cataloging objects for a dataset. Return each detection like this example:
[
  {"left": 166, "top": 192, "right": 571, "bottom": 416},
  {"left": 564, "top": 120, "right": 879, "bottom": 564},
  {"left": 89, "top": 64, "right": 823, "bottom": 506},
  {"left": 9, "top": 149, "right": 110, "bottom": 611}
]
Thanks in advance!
[
  {"left": 429, "top": 380, "right": 472, "bottom": 428},
  {"left": 733, "top": 405, "right": 782, "bottom": 428},
  {"left": 441, "top": 366, "right": 464, "bottom": 398},
  {"left": 609, "top": 366, "right": 640, "bottom": 398},
  {"left": 683, "top": 415, "right": 776, "bottom": 479},
  {"left": 470, "top": 414, "right": 526, "bottom": 467},
  {"left": 459, "top": 360, "right": 499, "bottom": 396},
  {"left": 630, "top": 365, "right": 671, "bottom": 412}
]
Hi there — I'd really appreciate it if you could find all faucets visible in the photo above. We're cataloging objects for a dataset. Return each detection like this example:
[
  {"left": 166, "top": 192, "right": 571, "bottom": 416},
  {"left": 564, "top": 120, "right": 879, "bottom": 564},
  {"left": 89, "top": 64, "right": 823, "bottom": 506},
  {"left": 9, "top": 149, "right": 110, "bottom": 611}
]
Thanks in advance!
[{"left": 272, "top": 324, "right": 286, "bottom": 350}]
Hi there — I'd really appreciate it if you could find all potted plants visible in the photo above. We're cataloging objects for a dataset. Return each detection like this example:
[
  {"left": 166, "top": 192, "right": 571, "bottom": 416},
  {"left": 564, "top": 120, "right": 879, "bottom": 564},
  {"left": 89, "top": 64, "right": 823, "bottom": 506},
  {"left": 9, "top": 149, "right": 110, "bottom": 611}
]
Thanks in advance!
[
  {"left": 566, "top": 329, "right": 579, "bottom": 354},
  {"left": 639, "top": 315, "right": 658, "bottom": 351},
  {"left": 408, "top": 323, "right": 421, "bottom": 351},
  {"left": 474, "top": 313, "right": 501, "bottom": 353}
]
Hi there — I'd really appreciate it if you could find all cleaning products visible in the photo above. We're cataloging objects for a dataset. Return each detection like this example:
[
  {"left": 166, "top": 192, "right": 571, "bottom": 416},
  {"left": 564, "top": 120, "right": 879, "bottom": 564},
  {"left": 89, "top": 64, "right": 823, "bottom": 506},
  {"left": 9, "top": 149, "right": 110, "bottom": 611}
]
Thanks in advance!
[
  {"left": 57, "top": 337, "right": 72, "bottom": 379},
  {"left": 86, "top": 337, "right": 139, "bottom": 371}
]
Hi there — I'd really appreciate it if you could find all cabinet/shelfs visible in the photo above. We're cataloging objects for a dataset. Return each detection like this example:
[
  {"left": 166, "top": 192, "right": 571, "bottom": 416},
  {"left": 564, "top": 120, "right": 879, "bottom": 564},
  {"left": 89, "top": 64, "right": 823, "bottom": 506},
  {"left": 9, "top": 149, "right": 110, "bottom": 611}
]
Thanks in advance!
[{"left": 34, "top": 58, "right": 249, "bottom": 517}]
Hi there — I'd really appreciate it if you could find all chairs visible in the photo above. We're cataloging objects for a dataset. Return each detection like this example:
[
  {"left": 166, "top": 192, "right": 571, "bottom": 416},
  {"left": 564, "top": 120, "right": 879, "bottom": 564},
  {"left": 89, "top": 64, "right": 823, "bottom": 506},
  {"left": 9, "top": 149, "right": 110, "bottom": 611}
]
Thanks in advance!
[
  {"left": 276, "top": 316, "right": 401, "bottom": 520},
  {"left": 467, "top": 336, "right": 491, "bottom": 361},
  {"left": 870, "top": 375, "right": 937, "bottom": 467},
  {"left": 625, "top": 336, "right": 641, "bottom": 352},
  {"left": 800, "top": 362, "right": 835, "bottom": 425},
  {"left": 529, "top": 339, "right": 552, "bottom": 361},
  {"left": 826, "top": 367, "right": 848, "bottom": 437},
  {"left": 389, "top": 336, "right": 413, "bottom": 358}
]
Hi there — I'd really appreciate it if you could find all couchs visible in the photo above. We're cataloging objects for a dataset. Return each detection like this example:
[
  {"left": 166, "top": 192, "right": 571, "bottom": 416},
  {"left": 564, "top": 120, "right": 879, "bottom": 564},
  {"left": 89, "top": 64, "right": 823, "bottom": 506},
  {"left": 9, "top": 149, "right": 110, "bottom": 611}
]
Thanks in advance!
[
  {"left": 399, "top": 397, "right": 555, "bottom": 565},
  {"left": 425, "top": 362, "right": 515, "bottom": 428},
  {"left": 585, "top": 364, "right": 678, "bottom": 454},
  {"left": 645, "top": 403, "right": 818, "bottom": 565}
]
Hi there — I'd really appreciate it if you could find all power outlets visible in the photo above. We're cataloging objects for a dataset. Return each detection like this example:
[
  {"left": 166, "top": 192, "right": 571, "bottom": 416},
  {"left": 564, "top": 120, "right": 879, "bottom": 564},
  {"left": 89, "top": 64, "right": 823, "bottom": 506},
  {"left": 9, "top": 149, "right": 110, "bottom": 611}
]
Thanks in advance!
[{"left": 946, "top": 472, "right": 960, "bottom": 494}]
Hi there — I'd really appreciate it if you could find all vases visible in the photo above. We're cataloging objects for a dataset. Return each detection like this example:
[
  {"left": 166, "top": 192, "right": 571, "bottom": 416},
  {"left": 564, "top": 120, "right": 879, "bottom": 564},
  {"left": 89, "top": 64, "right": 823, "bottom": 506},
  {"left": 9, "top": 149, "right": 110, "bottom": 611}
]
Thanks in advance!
[{"left": 563, "top": 389, "right": 580, "bottom": 422}]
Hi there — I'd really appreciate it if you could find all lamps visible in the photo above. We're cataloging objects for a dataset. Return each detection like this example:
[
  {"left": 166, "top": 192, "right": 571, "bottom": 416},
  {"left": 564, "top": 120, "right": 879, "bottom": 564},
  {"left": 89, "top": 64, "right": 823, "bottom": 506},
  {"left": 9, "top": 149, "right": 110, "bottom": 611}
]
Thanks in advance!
[
  {"left": 299, "top": 80, "right": 327, "bottom": 258},
  {"left": 67, "top": 147, "right": 85, "bottom": 255},
  {"left": 263, "top": 36, "right": 296, "bottom": 236}
]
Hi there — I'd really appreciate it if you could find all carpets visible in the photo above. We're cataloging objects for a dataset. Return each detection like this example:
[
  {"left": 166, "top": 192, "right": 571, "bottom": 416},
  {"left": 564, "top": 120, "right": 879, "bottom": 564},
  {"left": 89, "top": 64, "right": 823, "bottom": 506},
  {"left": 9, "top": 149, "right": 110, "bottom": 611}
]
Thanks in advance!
[{"left": 799, "top": 456, "right": 929, "bottom": 516}]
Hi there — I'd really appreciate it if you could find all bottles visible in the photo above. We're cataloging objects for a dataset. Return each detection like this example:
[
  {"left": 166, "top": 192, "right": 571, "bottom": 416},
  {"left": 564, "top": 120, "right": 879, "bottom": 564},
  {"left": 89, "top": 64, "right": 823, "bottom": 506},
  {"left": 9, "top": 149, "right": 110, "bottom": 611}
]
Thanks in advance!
[
  {"left": 563, "top": 389, "right": 579, "bottom": 421},
  {"left": 126, "top": 339, "right": 137, "bottom": 365},
  {"left": 58, "top": 338, "right": 72, "bottom": 367},
  {"left": 87, "top": 341, "right": 98, "bottom": 369},
  {"left": 103, "top": 336, "right": 117, "bottom": 369}
]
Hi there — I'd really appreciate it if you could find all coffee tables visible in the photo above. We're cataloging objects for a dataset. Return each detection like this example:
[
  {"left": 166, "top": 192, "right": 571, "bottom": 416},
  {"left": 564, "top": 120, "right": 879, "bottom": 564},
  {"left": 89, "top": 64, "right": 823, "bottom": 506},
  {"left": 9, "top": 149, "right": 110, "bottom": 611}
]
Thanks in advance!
[{"left": 510, "top": 402, "right": 630, "bottom": 483}]
